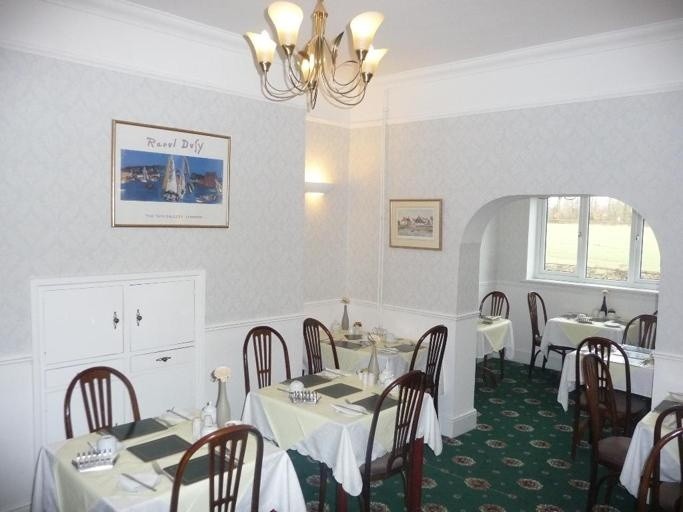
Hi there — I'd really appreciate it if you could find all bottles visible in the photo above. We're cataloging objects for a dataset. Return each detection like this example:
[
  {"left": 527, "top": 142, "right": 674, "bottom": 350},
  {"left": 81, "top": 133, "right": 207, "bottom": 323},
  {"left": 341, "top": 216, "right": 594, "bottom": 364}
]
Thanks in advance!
[
  {"left": 73, "top": 448, "right": 114, "bottom": 471},
  {"left": 330, "top": 319, "right": 400, "bottom": 352},
  {"left": 192, "top": 417, "right": 203, "bottom": 443},
  {"left": 199, "top": 401, "right": 218, "bottom": 425},
  {"left": 356, "top": 359, "right": 401, "bottom": 395},
  {"left": 288, "top": 389, "right": 319, "bottom": 406}
]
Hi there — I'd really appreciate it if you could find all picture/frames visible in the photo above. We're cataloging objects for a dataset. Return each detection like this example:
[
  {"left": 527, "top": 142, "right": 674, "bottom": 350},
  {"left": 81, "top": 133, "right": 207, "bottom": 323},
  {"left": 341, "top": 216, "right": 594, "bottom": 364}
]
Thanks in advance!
[
  {"left": 109, "top": 119, "right": 232, "bottom": 230},
  {"left": 388, "top": 198, "right": 442, "bottom": 250}
]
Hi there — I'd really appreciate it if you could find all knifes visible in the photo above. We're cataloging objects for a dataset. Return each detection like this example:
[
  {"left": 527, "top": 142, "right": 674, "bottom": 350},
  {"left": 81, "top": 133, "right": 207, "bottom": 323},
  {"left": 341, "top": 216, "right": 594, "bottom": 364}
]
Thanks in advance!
[
  {"left": 334, "top": 403, "right": 367, "bottom": 415},
  {"left": 120, "top": 471, "right": 156, "bottom": 492}
]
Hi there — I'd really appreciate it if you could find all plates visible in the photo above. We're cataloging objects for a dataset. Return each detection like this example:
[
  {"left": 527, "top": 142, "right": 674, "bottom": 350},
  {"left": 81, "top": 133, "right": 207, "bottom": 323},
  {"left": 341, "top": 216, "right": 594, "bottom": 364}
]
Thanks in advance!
[{"left": 480, "top": 315, "right": 502, "bottom": 322}]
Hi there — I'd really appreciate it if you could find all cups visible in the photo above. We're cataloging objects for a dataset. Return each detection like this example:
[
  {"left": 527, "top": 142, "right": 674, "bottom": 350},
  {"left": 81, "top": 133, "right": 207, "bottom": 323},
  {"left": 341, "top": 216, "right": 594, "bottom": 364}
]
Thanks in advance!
[
  {"left": 201, "top": 424, "right": 219, "bottom": 443},
  {"left": 561, "top": 306, "right": 632, "bottom": 327},
  {"left": 289, "top": 380, "right": 304, "bottom": 393}
]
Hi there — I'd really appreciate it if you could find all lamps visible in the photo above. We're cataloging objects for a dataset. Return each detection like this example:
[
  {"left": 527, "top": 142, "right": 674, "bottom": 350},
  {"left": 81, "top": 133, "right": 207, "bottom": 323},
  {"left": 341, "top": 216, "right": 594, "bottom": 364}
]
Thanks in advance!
[{"left": 242, "top": 0, "right": 387, "bottom": 109}]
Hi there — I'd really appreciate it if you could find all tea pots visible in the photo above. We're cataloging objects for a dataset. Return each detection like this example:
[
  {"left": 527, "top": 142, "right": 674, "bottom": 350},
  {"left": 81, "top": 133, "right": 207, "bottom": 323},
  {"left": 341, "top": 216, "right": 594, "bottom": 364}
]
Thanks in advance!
[{"left": 87, "top": 434, "right": 125, "bottom": 454}]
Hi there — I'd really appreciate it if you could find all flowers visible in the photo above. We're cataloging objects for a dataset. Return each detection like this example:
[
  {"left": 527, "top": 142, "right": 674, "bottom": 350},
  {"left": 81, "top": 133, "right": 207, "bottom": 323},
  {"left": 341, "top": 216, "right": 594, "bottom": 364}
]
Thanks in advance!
[
  {"left": 340, "top": 294, "right": 351, "bottom": 306},
  {"left": 366, "top": 333, "right": 382, "bottom": 345},
  {"left": 602, "top": 289, "right": 609, "bottom": 295},
  {"left": 211, "top": 366, "right": 232, "bottom": 384}
]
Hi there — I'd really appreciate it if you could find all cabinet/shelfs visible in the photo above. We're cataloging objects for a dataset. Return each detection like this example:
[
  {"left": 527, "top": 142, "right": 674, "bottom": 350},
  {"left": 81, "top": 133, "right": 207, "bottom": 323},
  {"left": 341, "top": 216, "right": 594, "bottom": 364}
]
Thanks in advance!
[{"left": 34, "top": 271, "right": 207, "bottom": 451}]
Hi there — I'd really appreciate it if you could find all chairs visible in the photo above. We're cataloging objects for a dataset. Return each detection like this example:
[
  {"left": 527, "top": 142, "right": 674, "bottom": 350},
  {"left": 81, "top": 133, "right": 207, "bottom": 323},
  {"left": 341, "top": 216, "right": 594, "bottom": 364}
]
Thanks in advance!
[
  {"left": 636, "top": 429, "right": 681, "bottom": 511},
  {"left": 582, "top": 354, "right": 633, "bottom": 511},
  {"left": 169, "top": 421, "right": 264, "bottom": 512},
  {"left": 527, "top": 291, "right": 576, "bottom": 387},
  {"left": 317, "top": 365, "right": 426, "bottom": 509},
  {"left": 239, "top": 322, "right": 291, "bottom": 398},
  {"left": 404, "top": 324, "right": 449, "bottom": 421},
  {"left": 572, "top": 336, "right": 651, "bottom": 461},
  {"left": 621, "top": 313, "right": 658, "bottom": 350},
  {"left": 480, "top": 291, "right": 511, "bottom": 385},
  {"left": 64, "top": 366, "right": 137, "bottom": 449},
  {"left": 301, "top": 317, "right": 340, "bottom": 374},
  {"left": 650, "top": 404, "right": 681, "bottom": 511}
]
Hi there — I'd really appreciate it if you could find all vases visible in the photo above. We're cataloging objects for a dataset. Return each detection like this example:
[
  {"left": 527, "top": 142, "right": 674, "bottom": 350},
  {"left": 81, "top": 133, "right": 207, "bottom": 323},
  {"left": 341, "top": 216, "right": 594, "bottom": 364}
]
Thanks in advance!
[
  {"left": 216, "top": 383, "right": 230, "bottom": 428},
  {"left": 341, "top": 306, "right": 349, "bottom": 330},
  {"left": 367, "top": 347, "right": 380, "bottom": 385},
  {"left": 600, "top": 297, "right": 608, "bottom": 316}
]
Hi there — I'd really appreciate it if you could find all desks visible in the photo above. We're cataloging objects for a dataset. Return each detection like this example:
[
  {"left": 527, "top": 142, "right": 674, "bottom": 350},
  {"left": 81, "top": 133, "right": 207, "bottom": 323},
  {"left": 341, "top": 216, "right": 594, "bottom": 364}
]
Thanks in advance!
[
  {"left": 620, "top": 393, "right": 682, "bottom": 503},
  {"left": 558, "top": 344, "right": 656, "bottom": 413},
  {"left": 540, "top": 313, "right": 643, "bottom": 352},
  {"left": 476, "top": 316, "right": 515, "bottom": 388}
]
